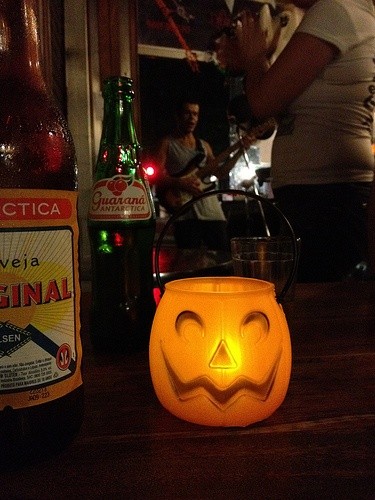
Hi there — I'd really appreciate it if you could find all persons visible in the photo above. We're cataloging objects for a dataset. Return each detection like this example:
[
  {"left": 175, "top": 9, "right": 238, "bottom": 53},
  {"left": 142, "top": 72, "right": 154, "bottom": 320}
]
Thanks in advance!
[
  {"left": 155, "top": 101, "right": 257, "bottom": 267},
  {"left": 227, "top": 1, "right": 375, "bottom": 282}
]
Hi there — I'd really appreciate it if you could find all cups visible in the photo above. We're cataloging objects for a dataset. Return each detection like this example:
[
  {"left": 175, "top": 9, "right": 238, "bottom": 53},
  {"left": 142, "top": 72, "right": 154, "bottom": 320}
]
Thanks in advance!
[{"left": 229, "top": 236, "right": 299, "bottom": 303}]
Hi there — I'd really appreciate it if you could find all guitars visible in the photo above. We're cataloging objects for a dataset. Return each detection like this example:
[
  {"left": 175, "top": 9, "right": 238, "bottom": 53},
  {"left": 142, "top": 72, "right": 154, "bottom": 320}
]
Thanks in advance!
[{"left": 154, "top": 117, "right": 277, "bottom": 214}]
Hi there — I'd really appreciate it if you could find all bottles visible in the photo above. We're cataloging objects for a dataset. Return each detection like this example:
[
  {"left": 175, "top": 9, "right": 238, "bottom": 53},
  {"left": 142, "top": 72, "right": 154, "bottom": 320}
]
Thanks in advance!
[
  {"left": 148, "top": 276, "right": 294, "bottom": 430},
  {"left": 89, "top": 74, "right": 157, "bottom": 357},
  {"left": 0, "top": 0, "right": 85, "bottom": 469}
]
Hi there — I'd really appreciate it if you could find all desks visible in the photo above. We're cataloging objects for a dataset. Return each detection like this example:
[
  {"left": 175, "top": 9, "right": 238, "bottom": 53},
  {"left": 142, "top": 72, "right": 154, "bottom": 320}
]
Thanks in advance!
[{"left": 0, "top": 278, "right": 375, "bottom": 500}]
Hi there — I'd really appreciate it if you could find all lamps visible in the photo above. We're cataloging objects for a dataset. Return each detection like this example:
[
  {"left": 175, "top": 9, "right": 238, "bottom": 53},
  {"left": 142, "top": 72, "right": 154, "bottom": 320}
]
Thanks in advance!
[{"left": 148, "top": 189, "right": 303, "bottom": 427}]
